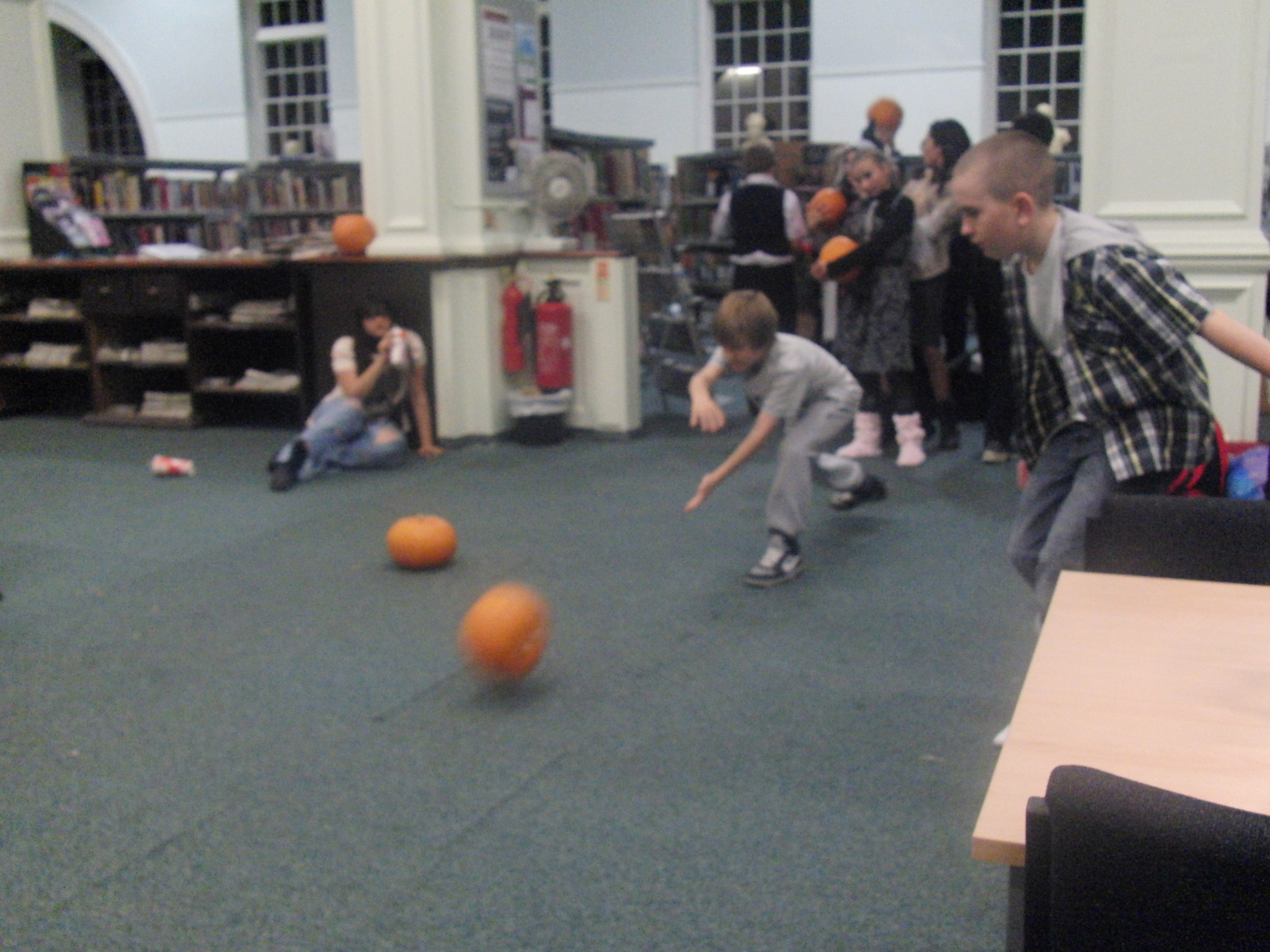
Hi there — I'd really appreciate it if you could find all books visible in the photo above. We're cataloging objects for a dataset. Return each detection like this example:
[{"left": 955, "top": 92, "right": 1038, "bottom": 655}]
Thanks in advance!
[
  {"left": 25, "top": 340, "right": 79, "bottom": 366},
  {"left": 597, "top": 147, "right": 735, "bottom": 355},
  {"left": 141, "top": 390, "right": 195, "bottom": 422},
  {"left": 234, "top": 365, "right": 296, "bottom": 393},
  {"left": 26, "top": 170, "right": 349, "bottom": 260},
  {"left": 223, "top": 295, "right": 289, "bottom": 326},
  {"left": 29, "top": 298, "right": 79, "bottom": 320},
  {"left": 141, "top": 343, "right": 192, "bottom": 363}
]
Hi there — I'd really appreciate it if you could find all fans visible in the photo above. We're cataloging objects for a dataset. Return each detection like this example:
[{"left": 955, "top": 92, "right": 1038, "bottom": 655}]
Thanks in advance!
[{"left": 520, "top": 149, "right": 592, "bottom": 253}]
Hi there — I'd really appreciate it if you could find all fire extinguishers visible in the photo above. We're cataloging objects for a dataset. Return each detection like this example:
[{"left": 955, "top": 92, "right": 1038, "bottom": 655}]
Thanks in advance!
[
  {"left": 503, "top": 273, "right": 534, "bottom": 372},
  {"left": 526, "top": 279, "right": 578, "bottom": 394}
]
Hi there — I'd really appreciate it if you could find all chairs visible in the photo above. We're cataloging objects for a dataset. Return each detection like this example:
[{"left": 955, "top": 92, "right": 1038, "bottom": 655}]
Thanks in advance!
[
  {"left": 613, "top": 211, "right": 711, "bottom": 416},
  {"left": 1081, "top": 496, "right": 1270, "bottom": 586},
  {"left": 1018, "top": 765, "right": 1269, "bottom": 952}
]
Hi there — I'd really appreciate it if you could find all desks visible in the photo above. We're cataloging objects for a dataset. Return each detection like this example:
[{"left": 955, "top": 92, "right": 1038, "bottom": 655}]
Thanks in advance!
[{"left": 975, "top": 569, "right": 1270, "bottom": 866}]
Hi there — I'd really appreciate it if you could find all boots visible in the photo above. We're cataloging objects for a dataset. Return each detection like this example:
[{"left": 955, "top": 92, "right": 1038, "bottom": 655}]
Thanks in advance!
[
  {"left": 891, "top": 412, "right": 928, "bottom": 466},
  {"left": 834, "top": 409, "right": 884, "bottom": 458}
]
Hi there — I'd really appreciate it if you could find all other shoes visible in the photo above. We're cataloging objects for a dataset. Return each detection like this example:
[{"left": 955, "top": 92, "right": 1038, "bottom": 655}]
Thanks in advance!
[{"left": 267, "top": 463, "right": 297, "bottom": 492}]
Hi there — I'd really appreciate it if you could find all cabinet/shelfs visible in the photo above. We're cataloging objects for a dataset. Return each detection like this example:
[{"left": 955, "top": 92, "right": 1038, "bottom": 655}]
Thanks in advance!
[
  {"left": 2, "top": 252, "right": 520, "bottom": 442},
  {"left": 552, "top": 127, "right": 841, "bottom": 389},
  {"left": 58, "top": 154, "right": 363, "bottom": 250}
]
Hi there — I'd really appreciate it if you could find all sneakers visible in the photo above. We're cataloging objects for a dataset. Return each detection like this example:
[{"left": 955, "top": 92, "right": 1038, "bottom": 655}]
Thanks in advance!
[
  {"left": 830, "top": 475, "right": 887, "bottom": 509},
  {"left": 744, "top": 549, "right": 808, "bottom": 588}
]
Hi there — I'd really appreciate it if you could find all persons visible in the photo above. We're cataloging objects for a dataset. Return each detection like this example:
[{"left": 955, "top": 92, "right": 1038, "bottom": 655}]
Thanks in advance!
[
  {"left": 807, "top": 103, "right": 1062, "bottom": 468},
  {"left": 681, "top": 291, "right": 886, "bottom": 585},
  {"left": 265, "top": 293, "right": 444, "bottom": 492},
  {"left": 955, "top": 135, "right": 1270, "bottom": 634},
  {"left": 706, "top": 146, "right": 806, "bottom": 427}
]
